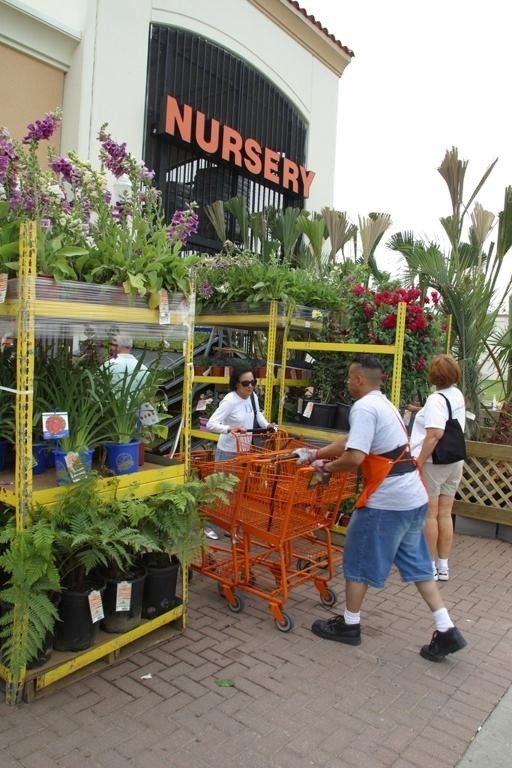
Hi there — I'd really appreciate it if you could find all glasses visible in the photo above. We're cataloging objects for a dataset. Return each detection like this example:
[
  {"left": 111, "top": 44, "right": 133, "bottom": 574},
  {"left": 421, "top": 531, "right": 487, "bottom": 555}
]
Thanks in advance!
[{"left": 239, "top": 380, "right": 257, "bottom": 386}]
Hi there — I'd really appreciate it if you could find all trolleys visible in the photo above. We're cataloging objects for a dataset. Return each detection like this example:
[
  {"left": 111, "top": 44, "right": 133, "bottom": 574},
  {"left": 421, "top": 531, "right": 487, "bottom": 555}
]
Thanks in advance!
[
  {"left": 227, "top": 423, "right": 359, "bottom": 569},
  {"left": 160, "top": 447, "right": 321, "bottom": 614},
  {"left": 196, "top": 456, "right": 350, "bottom": 634}
]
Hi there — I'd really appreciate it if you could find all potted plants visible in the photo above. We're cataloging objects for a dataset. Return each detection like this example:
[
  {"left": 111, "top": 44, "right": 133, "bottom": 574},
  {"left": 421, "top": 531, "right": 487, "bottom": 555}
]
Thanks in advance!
[
  {"left": 181, "top": 237, "right": 452, "bottom": 538},
  {"left": 2, "top": 107, "right": 240, "bottom": 711}
]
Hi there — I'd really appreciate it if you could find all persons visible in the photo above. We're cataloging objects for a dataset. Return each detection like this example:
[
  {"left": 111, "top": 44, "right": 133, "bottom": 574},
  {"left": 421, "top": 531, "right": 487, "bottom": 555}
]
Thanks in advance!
[
  {"left": 410, "top": 353, "right": 466, "bottom": 581},
  {"left": 291, "top": 357, "right": 467, "bottom": 662},
  {"left": 204, "top": 367, "right": 278, "bottom": 540},
  {"left": 93, "top": 332, "right": 150, "bottom": 412}
]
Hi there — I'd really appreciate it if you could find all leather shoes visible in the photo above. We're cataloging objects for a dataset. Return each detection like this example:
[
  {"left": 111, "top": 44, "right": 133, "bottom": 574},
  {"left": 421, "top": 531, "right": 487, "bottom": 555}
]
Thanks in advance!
[
  {"left": 420, "top": 628, "right": 466, "bottom": 661},
  {"left": 312, "top": 619, "right": 362, "bottom": 646}
]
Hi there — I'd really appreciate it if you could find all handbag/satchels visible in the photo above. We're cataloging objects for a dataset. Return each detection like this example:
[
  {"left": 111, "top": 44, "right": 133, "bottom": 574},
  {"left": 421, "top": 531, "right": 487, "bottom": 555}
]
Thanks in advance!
[{"left": 432, "top": 419, "right": 466, "bottom": 464}]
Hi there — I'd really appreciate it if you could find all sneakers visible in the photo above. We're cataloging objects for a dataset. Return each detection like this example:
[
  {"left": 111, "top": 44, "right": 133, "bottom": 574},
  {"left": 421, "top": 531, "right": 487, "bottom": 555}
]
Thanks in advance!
[
  {"left": 432, "top": 568, "right": 438, "bottom": 581},
  {"left": 437, "top": 567, "right": 449, "bottom": 581}
]
